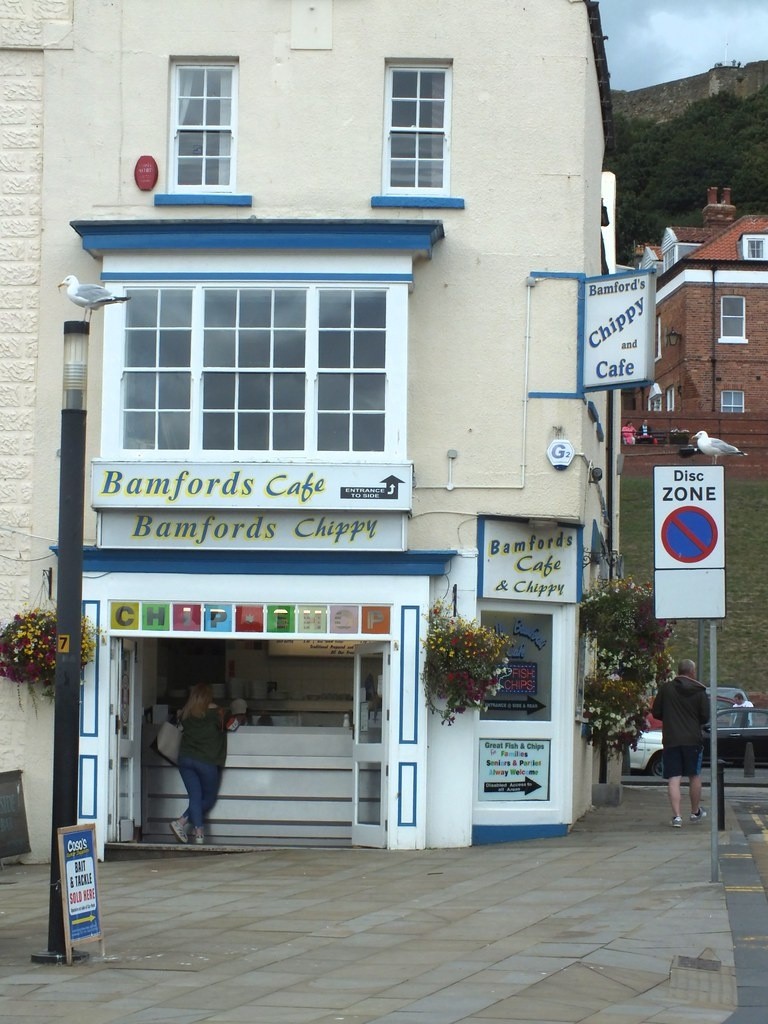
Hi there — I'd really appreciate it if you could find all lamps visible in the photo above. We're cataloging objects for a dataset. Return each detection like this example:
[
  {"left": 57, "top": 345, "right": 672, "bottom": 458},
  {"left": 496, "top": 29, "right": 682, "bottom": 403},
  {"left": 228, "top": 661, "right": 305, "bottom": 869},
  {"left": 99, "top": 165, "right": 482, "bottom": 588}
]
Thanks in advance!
[{"left": 666, "top": 327, "right": 682, "bottom": 346}]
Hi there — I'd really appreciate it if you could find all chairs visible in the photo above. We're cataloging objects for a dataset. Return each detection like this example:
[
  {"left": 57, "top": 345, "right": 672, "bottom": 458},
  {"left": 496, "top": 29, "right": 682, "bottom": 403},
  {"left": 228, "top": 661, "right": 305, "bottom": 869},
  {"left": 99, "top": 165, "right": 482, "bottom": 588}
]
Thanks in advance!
[{"left": 733, "top": 717, "right": 750, "bottom": 727}]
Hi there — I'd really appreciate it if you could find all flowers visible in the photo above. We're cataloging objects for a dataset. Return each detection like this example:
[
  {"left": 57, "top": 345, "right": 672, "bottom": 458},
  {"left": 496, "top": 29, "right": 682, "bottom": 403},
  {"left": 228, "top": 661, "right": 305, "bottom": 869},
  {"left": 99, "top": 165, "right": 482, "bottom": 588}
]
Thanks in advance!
[
  {"left": 418, "top": 596, "right": 514, "bottom": 726},
  {"left": 578, "top": 574, "right": 677, "bottom": 767},
  {"left": 0, "top": 598, "right": 108, "bottom": 721}
]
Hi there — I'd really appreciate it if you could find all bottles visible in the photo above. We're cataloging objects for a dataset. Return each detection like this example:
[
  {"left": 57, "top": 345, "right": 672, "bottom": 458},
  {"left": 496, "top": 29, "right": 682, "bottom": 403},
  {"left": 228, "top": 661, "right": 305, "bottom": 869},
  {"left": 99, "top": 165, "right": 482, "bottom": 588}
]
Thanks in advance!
[{"left": 343, "top": 713, "right": 351, "bottom": 727}]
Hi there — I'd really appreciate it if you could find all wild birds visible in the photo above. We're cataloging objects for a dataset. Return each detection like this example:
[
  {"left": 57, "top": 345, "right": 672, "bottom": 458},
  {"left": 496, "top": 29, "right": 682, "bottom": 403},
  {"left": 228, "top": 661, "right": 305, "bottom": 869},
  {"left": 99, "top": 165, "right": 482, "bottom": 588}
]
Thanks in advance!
[
  {"left": 691, "top": 430, "right": 749, "bottom": 465},
  {"left": 57, "top": 275, "right": 133, "bottom": 323}
]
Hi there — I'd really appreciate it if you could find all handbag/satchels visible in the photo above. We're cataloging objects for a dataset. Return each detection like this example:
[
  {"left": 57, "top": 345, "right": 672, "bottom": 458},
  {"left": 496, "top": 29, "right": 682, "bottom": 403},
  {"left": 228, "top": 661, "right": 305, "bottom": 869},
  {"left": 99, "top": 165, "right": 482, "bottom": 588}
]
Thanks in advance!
[{"left": 150, "top": 710, "right": 185, "bottom": 766}]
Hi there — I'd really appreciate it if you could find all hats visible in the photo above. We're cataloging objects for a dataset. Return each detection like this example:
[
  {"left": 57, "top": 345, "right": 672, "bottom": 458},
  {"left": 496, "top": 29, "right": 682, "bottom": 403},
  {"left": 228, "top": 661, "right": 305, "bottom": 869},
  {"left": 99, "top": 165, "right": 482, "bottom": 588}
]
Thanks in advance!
[{"left": 231, "top": 699, "right": 246, "bottom": 716}]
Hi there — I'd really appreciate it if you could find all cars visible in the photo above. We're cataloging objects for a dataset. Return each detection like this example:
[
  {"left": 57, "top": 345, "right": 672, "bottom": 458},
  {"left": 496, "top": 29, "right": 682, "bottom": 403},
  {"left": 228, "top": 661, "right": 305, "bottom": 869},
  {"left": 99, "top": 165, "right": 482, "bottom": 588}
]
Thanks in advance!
[
  {"left": 639, "top": 687, "right": 764, "bottom": 732},
  {"left": 628, "top": 729, "right": 664, "bottom": 777},
  {"left": 701, "top": 708, "right": 768, "bottom": 766}
]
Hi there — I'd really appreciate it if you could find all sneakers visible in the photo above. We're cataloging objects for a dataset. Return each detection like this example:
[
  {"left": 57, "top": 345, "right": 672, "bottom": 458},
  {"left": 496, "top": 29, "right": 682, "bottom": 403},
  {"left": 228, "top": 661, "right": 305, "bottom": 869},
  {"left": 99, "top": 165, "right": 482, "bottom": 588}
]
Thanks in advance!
[
  {"left": 689, "top": 808, "right": 707, "bottom": 823},
  {"left": 672, "top": 816, "right": 682, "bottom": 827}
]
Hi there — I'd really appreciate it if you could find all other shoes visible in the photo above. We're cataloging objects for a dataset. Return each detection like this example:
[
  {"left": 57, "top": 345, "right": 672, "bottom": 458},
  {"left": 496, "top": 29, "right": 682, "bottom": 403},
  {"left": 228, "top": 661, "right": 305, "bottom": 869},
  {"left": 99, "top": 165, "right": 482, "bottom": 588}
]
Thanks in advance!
[
  {"left": 193, "top": 833, "right": 204, "bottom": 844},
  {"left": 170, "top": 821, "right": 188, "bottom": 843}
]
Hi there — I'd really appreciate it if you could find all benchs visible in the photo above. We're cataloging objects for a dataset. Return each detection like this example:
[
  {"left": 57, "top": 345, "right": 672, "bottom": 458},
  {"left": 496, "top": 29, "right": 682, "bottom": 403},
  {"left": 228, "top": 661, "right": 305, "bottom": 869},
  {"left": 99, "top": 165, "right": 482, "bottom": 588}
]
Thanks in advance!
[{"left": 621, "top": 432, "right": 668, "bottom": 445}]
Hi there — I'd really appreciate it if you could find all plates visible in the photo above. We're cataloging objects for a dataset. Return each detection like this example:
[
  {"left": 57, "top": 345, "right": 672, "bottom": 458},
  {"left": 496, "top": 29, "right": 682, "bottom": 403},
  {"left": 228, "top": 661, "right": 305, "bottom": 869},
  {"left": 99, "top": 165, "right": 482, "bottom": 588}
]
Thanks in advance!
[{"left": 209, "top": 678, "right": 290, "bottom": 700}]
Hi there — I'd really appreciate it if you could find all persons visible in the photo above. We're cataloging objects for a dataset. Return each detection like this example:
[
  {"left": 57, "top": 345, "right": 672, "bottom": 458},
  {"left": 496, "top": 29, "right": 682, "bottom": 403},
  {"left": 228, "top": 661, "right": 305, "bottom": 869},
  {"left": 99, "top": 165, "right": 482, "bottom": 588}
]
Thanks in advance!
[
  {"left": 622, "top": 420, "right": 652, "bottom": 445},
  {"left": 651, "top": 660, "right": 710, "bottom": 826},
  {"left": 170, "top": 683, "right": 250, "bottom": 845},
  {"left": 729, "top": 693, "right": 754, "bottom": 727}
]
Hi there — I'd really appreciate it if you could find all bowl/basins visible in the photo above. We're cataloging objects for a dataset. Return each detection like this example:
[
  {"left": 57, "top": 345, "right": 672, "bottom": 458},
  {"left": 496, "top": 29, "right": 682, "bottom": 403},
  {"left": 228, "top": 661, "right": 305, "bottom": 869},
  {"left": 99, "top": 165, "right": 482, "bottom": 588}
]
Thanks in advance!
[{"left": 292, "top": 692, "right": 353, "bottom": 701}]
